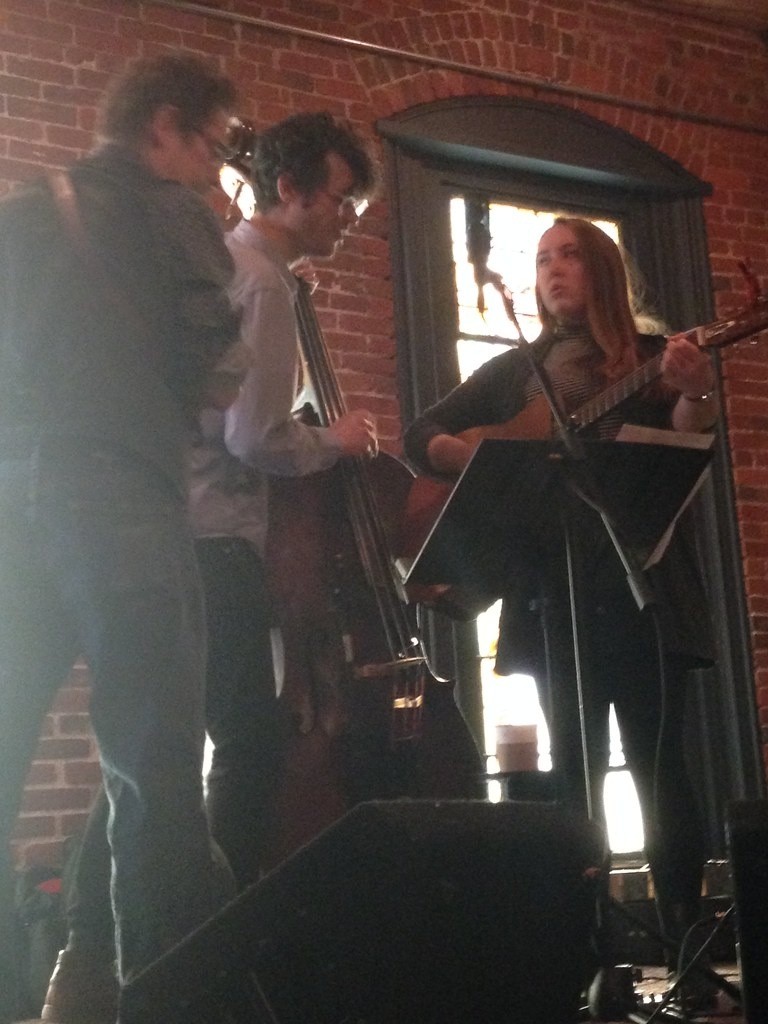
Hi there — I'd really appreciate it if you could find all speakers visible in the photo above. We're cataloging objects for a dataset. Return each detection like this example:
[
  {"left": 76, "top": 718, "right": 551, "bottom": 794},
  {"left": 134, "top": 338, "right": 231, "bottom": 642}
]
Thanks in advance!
[{"left": 116, "top": 800, "right": 603, "bottom": 1024}]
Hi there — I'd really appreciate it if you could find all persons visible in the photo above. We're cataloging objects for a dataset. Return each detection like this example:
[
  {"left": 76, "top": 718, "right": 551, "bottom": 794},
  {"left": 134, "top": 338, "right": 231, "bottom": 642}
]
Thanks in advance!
[
  {"left": 0, "top": 43, "right": 256, "bottom": 1023},
  {"left": 39, "top": 109, "right": 380, "bottom": 1024},
  {"left": 401, "top": 213, "right": 727, "bottom": 1015}
]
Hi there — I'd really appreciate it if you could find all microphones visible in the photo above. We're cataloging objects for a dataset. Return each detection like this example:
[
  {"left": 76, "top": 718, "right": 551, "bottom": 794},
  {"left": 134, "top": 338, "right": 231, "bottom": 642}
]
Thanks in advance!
[{"left": 462, "top": 191, "right": 491, "bottom": 312}]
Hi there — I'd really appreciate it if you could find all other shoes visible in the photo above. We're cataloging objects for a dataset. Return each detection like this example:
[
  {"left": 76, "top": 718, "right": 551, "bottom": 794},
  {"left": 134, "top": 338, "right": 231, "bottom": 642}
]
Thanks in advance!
[{"left": 41, "top": 948, "right": 121, "bottom": 1023}]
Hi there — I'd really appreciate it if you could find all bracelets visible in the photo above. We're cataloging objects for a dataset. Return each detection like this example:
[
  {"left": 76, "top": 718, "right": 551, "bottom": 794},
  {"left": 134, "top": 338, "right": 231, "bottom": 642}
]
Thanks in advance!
[{"left": 682, "top": 389, "right": 720, "bottom": 402}]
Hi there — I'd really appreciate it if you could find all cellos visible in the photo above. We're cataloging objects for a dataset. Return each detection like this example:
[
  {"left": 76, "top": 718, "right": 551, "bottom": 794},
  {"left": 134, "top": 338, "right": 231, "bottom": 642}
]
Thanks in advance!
[{"left": 191, "top": 117, "right": 487, "bottom": 888}]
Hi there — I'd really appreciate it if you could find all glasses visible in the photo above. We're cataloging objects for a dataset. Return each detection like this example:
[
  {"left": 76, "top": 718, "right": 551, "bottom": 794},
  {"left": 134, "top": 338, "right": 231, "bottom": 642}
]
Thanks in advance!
[
  {"left": 320, "top": 179, "right": 370, "bottom": 227},
  {"left": 191, "top": 118, "right": 257, "bottom": 171}
]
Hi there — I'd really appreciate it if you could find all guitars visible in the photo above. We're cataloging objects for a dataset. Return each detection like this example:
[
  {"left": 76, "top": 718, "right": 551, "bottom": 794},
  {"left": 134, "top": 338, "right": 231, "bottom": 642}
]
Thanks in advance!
[{"left": 399, "top": 283, "right": 767, "bottom": 622}]
